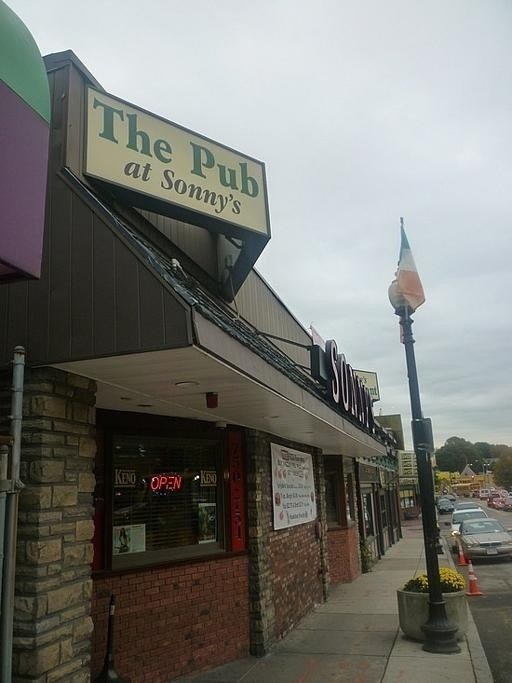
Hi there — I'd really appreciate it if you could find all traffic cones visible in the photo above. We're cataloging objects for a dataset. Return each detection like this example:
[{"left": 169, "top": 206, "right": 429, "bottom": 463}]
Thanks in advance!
[{"left": 465, "top": 560, "right": 483, "bottom": 596}]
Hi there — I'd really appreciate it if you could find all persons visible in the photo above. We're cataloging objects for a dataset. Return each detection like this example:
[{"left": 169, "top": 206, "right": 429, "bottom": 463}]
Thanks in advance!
[{"left": 117, "top": 527, "right": 131, "bottom": 553}]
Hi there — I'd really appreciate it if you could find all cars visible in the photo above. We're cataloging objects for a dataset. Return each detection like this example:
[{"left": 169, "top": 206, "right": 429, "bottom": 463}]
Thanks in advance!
[
  {"left": 436, "top": 494, "right": 456, "bottom": 514},
  {"left": 470, "top": 487, "right": 512, "bottom": 511},
  {"left": 445, "top": 502, "right": 512, "bottom": 565}
]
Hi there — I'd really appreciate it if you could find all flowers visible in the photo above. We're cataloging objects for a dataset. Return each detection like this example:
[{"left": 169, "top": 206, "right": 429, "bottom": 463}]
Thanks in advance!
[{"left": 403, "top": 569, "right": 467, "bottom": 594}]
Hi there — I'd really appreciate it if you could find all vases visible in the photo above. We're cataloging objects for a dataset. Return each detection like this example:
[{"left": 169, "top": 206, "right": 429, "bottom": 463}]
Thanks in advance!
[{"left": 395, "top": 588, "right": 468, "bottom": 645}]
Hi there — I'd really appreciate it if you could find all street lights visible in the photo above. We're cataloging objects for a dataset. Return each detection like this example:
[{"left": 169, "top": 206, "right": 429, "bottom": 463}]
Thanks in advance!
[{"left": 388, "top": 261, "right": 462, "bottom": 653}]
